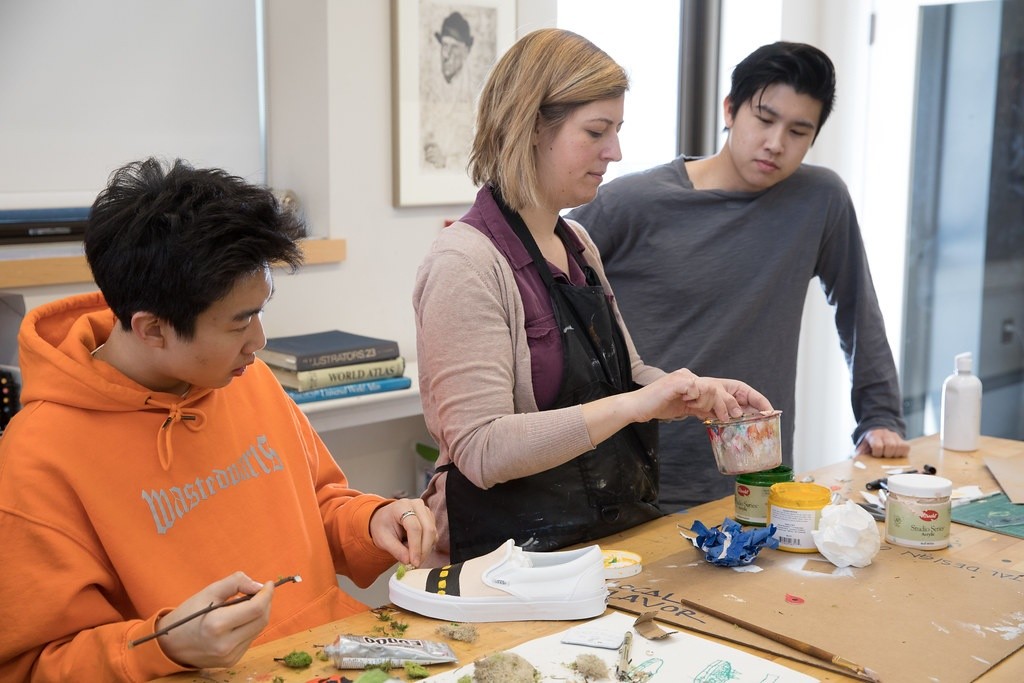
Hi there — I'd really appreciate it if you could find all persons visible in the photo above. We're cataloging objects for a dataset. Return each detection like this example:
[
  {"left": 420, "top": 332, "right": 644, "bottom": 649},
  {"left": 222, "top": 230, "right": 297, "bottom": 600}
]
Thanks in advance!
[
  {"left": 561, "top": 40, "right": 911, "bottom": 514},
  {"left": 410, "top": 24, "right": 772, "bottom": 559},
  {"left": 0, "top": 158, "right": 442, "bottom": 683}
]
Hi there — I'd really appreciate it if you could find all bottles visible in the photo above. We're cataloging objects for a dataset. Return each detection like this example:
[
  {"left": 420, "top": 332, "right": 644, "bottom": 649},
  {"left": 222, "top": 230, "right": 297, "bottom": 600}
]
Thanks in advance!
[{"left": 940, "top": 353, "right": 984, "bottom": 451}]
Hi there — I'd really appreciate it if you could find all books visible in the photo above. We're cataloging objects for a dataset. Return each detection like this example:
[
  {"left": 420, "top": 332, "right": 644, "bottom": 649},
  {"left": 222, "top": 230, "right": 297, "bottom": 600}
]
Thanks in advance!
[{"left": 251, "top": 328, "right": 414, "bottom": 405}]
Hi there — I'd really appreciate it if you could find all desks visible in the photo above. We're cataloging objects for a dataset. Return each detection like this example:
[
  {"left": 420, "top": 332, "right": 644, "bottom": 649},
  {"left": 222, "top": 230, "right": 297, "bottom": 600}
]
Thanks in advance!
[
  {"left": 144, "top": 429, "right": 1024, "bottom": 683},
  {"left": 296, "top": 361, "right": 438, "bottom": 607}
]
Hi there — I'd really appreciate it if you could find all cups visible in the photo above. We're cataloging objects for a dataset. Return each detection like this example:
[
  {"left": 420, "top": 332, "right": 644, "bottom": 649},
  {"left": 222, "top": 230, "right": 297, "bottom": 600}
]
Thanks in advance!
[{"left": 704, "top": 410, "right": 782, "bottom": 476}]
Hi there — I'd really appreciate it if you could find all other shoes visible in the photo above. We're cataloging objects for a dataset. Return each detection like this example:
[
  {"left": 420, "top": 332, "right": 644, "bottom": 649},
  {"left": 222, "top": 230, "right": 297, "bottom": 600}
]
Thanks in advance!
[{"left": 389, "top": 538, "right": 608, "bottom": 623}]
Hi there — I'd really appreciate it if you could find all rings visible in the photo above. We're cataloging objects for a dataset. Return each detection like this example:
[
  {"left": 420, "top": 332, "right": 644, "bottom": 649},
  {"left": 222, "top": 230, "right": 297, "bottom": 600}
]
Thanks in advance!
[{"left": 398, "top": 510, "right": 416, "bottom": 523}]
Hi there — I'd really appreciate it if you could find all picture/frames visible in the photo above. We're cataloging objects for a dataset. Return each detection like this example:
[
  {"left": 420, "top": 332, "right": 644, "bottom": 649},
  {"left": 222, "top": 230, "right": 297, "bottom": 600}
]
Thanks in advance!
[{"left": 389, "top": 0, "right": 520, "bottom": 210}]
